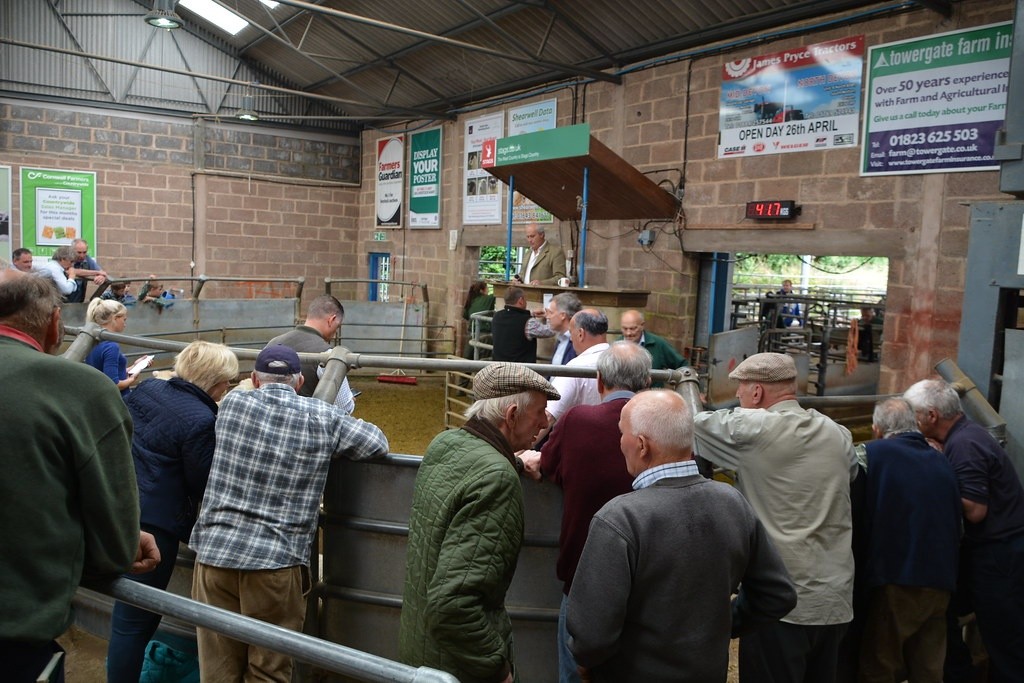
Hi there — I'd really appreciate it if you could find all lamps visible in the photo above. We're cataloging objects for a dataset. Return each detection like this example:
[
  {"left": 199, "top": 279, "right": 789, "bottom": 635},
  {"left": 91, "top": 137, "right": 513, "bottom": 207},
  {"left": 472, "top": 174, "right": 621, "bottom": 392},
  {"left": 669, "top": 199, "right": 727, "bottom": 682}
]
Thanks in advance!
[{"left": 143, "top": 0, "right": 185, "bottom": 30}]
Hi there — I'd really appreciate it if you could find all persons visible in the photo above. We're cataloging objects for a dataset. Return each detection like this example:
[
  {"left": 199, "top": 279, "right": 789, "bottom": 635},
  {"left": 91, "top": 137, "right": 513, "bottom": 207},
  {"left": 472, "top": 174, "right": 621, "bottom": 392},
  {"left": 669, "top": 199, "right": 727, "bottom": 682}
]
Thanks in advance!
[
  {"left": 776, "top": 280, "right": 803, "bottom": 354},
  {"left": 529, "top": 309, "right": 611, "bottom": 452},
  {"left": 616, "top": 309, "right": 708, "bottom": 405},
  {"left": 511, "top": 224, "right": 566, "bottom": 286},
  {"left": 265, "top": 295, "right": 357, "bottom": 417},
  {"left": 564, "top": 387, "right": 798, "bottom": 683},
  {"left": 455, "top": 281, "right": 495, "bottom": 396},
  {"left": 690, "top": 353, "right": 859, "bottom": 683},
  {"left": 84, "top": 297, "right": 153, "bottom": 397},
  {"left": 187, "top": 345, "right": 389, "bottom": 683},
  {"left": 0, "top": 269, "right": 160, "bottom": 683},
  {"left": 539, "top": 340, "right": 653, "bottom": 683},
  {"left": 853, "top": 396, "right": 965, "bottom": 683},
  {"left": 545, "top": 292, "right": 582, "bottom": 384},
  {"left": 905, "top": 378, "right": 1024, "bottom": 683},
  {"left": 401, "top": 362, "right": 562, "bottom": 683},
  {"left": 491, "top": 287, "right": 556, "bottom": 363},
  {"left": 763, "top": 291, "right": 785, "bottom": 348},
  {"left": 105, "top": 342, "right": 240, "bottom": 683},
  {"left": 0, "top": 239, "right": 166, "bottom": 306}
]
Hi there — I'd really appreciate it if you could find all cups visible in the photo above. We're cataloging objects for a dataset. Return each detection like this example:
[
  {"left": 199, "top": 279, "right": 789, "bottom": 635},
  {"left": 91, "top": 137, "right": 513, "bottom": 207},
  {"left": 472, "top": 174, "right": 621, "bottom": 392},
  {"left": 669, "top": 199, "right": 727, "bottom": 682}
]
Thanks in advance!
[{"left": 557, "top": 278, "right": 570, "bottom": 288}]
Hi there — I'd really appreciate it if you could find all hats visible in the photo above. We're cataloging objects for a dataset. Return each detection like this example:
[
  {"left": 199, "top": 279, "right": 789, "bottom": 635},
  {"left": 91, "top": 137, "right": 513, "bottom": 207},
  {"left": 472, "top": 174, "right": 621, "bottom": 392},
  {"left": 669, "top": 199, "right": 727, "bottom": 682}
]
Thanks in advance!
[
  {"left": 254, "top": 343, "right": 301, "bottom": 374},
  {"left": 728, "top": 352, "right": 798, "bottom": 383},
  {"left": 472, "top": 362, "right": 561, "bottom": 401}
]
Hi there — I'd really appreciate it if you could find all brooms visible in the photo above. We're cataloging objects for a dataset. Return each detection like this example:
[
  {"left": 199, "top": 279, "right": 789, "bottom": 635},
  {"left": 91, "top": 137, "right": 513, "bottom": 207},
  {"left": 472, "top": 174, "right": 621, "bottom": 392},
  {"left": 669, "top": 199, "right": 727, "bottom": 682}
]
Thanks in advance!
[{"left": 376, "top": 287, "right": 418, "bottom": 385}]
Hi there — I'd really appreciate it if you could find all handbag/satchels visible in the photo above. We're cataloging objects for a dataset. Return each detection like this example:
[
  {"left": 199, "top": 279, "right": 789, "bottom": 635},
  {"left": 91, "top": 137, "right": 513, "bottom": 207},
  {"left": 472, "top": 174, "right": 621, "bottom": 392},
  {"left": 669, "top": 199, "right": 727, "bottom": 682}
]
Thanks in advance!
[{"left": 140, "top": 639, "right": 201, "bottom": 683}]
